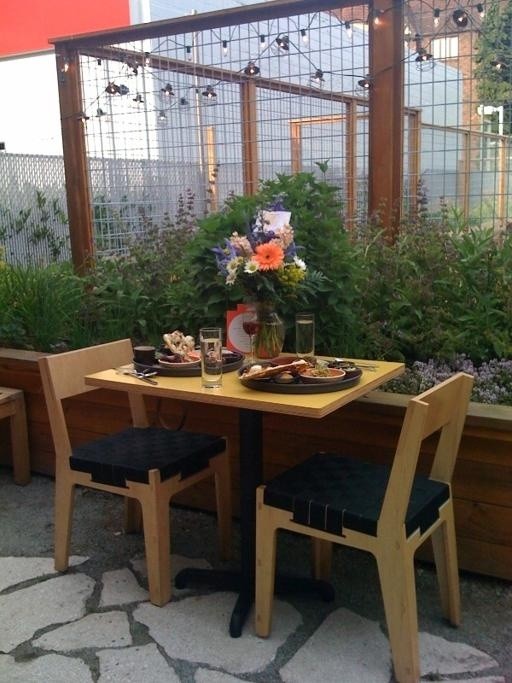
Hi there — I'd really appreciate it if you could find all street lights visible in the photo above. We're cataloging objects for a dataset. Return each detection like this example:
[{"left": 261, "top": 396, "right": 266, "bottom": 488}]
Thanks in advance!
[{"left": 476, "top": 104, "right": 506, "bottom": 223}]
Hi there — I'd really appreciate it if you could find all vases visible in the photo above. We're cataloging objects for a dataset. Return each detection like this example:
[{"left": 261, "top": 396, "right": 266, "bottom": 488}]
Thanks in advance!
[{"left": 246, "top": 305, "right": 285, "bottom": 359}]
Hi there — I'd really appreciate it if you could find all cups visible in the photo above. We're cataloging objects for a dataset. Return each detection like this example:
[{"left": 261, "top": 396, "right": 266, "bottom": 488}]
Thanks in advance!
[
  {"left": 293, "top": 312, "right": 318, "bottom": 360},
  {"left": 200, "top": 325, "right": 223, "bottom": 390}
]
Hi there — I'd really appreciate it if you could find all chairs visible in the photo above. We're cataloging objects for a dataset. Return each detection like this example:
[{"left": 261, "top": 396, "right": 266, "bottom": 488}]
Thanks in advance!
[
  {"left": 254, "top": 371, "right": 474, "bottom": 683},
  {"left": 37, "top": 336, "right": 234, "bottom": 609},
  {"left": 0, "top": 384, "right": 34, "bottom": 487}
]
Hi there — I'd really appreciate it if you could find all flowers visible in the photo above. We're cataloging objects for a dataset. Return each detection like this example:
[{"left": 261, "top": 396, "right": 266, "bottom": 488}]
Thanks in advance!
[{"left": 212, "top": 192, "right": 329, "bottom": 305}]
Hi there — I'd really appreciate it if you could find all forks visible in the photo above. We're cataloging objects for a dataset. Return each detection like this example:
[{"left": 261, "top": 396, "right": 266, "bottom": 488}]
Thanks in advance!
[{"left": 107, "top": 365, "right": 158, "bottom": 386}]
